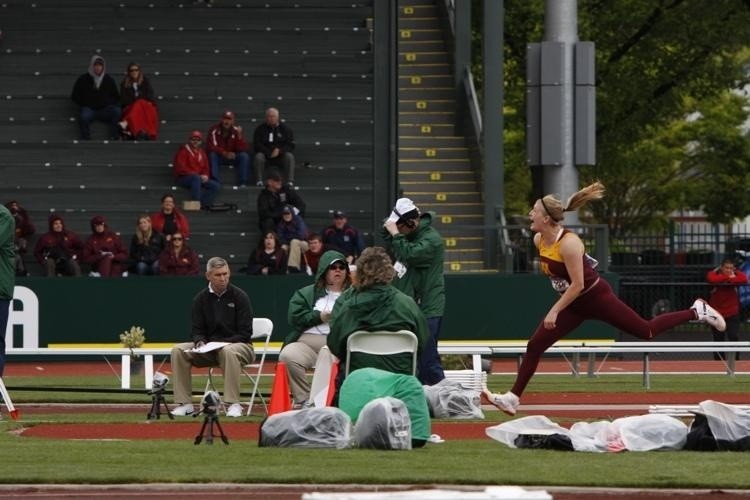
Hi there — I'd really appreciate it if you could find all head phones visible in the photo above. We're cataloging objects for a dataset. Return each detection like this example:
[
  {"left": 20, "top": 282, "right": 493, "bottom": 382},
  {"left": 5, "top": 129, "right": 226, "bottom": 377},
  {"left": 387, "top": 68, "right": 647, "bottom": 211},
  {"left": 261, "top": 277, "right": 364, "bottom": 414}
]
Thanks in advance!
[{"left": 392, "top": 207, "right": 415, "bottom": 228}]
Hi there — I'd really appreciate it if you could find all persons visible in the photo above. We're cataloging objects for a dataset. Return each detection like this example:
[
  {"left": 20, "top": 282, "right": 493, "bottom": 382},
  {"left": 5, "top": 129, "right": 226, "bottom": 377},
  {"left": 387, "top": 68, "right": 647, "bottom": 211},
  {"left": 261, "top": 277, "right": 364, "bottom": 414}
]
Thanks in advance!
[
  {"left": 6, "top": 193, "right": 200, "bottom": 278},
  {"left": 481, "top": 176, "right": 727, "bottom": 417},
  {"left": 734, "top": 258, "right": 750, "bottom": 324},
  {"left": 175, "top": 130, "right": 222, "bottom": 210},
  {"left": 71, "top": 55, "right": 122, "bottom": 140},
  {"left": 206, "top": 110, "right": 251, "bottom": 189},
  {"left": 0, "top": 204, "right": 16, "bottom": 378},
  {"left": 169, "top": 256, "right": 256, "bottom": 418},
  {"left": 251, "top": 107, "right": 296, "bottom": 187},
  {"left": 705, "top": 259, "right": 748, "bottom": 360},
  {"left": 118, "top": 62, "right": 160, "bottom": 141}
]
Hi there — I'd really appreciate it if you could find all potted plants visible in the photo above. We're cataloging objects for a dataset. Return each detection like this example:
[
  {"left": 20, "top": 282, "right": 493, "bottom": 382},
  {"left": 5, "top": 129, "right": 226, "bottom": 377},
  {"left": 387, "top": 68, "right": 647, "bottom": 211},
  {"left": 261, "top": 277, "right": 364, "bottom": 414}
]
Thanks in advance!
[{"left": 119, "top": 323, "right": 147, "bottom": 349}]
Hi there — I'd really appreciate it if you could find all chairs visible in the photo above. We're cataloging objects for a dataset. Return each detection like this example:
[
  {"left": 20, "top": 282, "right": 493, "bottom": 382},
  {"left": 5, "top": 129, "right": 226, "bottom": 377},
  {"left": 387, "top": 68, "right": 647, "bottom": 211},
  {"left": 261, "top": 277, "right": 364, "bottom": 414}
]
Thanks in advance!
[
  {"left": 342, "top": 326, "right": 418, "bottom": 382},
  {"left": 197, "top": 314, "right": 274, "bottom": 417}
]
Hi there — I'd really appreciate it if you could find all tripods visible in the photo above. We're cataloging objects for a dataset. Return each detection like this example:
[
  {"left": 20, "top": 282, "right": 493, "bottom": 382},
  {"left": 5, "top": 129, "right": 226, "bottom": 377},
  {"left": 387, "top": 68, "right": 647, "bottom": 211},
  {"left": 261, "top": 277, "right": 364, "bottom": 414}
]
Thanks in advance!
[
  {"left": 148, "top": 388, "right": 173, "bottom": 419},
  {"left": 195, "top": 414, "right": 229, "bottom": 445}
]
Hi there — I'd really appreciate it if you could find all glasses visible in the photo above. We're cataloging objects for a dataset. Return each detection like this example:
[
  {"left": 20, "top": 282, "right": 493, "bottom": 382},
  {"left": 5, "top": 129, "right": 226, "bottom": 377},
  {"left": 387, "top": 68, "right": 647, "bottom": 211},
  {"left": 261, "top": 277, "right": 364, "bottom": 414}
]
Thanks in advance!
[
  {"left": 191, "top": 137, "right": 201, "bottom": 142},
  {"left": 328, "top": 264, "right": 346, "bottom": 271},
  {"left": 172, "top": 236, "right": 183, "bottom": 242},
  {"left": 129, "top": 68, "right": 139, "bottom": 73}
]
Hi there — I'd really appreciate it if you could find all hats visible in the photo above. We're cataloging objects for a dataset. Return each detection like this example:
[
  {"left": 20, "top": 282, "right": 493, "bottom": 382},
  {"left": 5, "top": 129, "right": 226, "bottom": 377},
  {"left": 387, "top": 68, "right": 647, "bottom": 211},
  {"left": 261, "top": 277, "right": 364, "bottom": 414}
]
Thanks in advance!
[
  {"left": 260, "top": 165, "right": 284, "bottom": 181},
  {"left": 382, "top": 195, "right": 416, "bottom": 226},
  {"left": 333, "top": 209, "right": 347, "bottom": 219},
  {"left": 221, "top": 109, "right": 236, "bottom": 120},
  {"left": 280, "top": 205, "right": 292, "bottom": 213},
  {"left": 190, "top": 130, "right": 203, "bottom": 141}
]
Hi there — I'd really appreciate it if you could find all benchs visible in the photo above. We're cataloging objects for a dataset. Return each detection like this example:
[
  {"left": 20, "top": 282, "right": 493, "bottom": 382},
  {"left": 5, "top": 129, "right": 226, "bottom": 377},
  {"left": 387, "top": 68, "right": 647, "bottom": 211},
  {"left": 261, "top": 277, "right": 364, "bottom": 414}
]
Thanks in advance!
[{"left": 0, "top": 1, "right": 374, "bottom": 277}]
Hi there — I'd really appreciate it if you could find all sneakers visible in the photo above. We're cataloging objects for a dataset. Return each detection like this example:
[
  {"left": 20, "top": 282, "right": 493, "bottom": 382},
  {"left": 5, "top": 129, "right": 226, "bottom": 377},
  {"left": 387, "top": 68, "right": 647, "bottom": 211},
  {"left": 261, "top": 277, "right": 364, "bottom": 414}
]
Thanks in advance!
[
  {"left": 168, "top": 402, "right": 195, "bottom": 418},
  {"left": 225, "top": 402, "right": 245, "bottom": 418},
  {"left": 287, "top": 181, "right": 300, "bottom": 192},
  {"left": 689, "top": 298, "right": 729, "bottom": 334},
  {"left": 255, "top": 180, "right": 266, "bottom": 189},
  {"left": 480, "top": 387, "right": 522, "bottom": 417}
]
